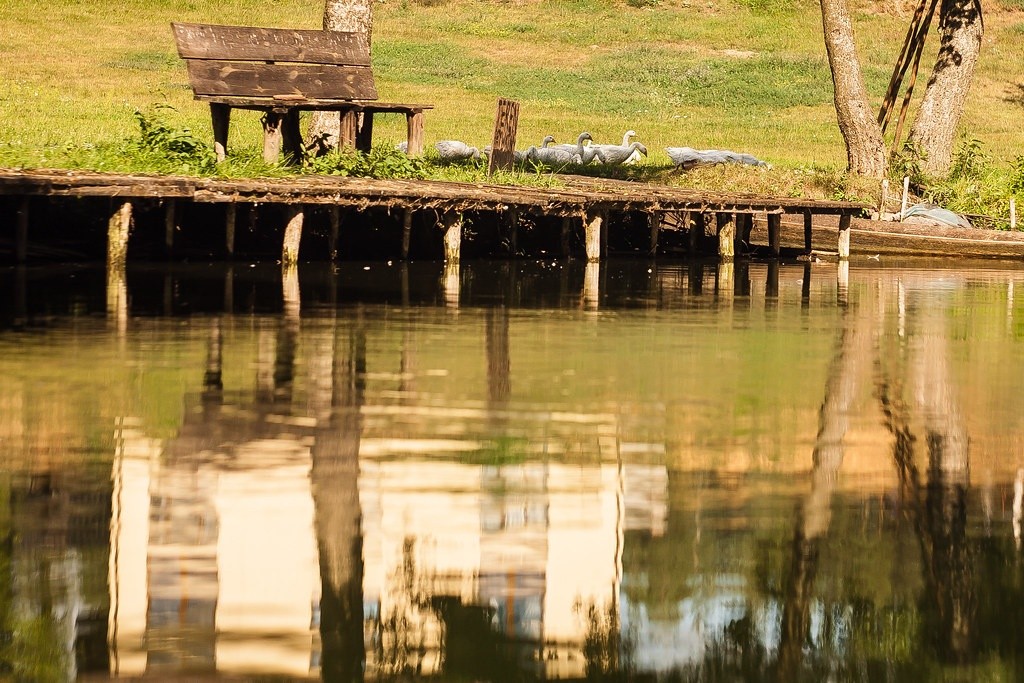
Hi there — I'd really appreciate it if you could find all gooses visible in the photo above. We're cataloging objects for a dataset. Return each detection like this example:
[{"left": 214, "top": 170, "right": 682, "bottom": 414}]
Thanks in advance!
[
  {"left": 484, "top": 129, "right": 648, "bottom": 175},
  {"left": 435, "top": 141, "right": 482, "bottom": 167},
  {"left": 395, "top": 141, "right": 407, "bottom": 155},
  {"left": 664, "top": 146, "right": 768, "bottom": 176}
]
